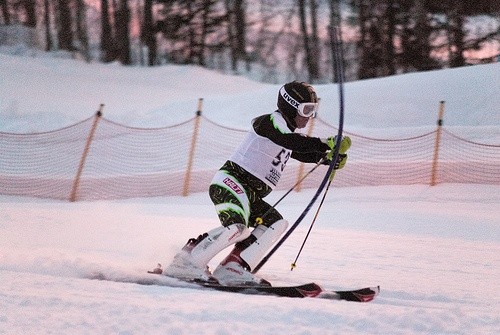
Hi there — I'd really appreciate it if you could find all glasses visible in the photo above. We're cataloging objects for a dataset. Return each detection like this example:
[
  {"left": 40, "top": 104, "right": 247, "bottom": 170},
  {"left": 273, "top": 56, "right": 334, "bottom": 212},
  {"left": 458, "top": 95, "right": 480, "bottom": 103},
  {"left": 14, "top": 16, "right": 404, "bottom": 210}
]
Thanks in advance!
[{"left": 297, "top": 103, "right": 320, "bottom": 118}]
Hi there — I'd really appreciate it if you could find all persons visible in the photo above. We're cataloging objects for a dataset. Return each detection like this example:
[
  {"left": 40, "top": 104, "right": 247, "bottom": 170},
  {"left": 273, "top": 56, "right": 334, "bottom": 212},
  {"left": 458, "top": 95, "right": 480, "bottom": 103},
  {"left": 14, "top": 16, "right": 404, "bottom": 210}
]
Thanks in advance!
[{"left": 159, "top": 80, "right": 352, "bottom": 289}]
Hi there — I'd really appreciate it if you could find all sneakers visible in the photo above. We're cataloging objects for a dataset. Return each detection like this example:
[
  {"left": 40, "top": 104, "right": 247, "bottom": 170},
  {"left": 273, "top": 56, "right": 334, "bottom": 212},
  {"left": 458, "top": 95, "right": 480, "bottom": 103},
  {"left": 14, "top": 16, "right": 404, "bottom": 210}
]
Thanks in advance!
[
  {"left": 162, "top": 251, "right": 214, "bottom": 283},
  {"left": 211, "top": 261, "right": 260, "bottom": 287}
]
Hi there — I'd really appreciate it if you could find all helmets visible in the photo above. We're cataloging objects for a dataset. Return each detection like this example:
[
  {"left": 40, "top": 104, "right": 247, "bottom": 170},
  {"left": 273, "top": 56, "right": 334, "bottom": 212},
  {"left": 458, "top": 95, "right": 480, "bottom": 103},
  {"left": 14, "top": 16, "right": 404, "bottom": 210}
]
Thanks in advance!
[{"left": 277, "top": 80, "right": 317, "bottom": 118}]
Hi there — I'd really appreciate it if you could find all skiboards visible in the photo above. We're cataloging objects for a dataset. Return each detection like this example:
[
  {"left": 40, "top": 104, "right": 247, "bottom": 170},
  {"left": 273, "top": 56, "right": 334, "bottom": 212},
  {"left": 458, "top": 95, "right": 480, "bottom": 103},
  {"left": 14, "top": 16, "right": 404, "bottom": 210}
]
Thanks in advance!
[{"left": 84, "top": 264, "right": 379, "bottom": 302}]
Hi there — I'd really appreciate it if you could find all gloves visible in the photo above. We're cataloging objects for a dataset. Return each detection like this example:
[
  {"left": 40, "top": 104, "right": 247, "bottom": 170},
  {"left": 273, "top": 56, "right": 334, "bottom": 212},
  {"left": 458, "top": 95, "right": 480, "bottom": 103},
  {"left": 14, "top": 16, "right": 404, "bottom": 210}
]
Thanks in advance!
[
  {"left": 327, "top": 151, "right": 348, "bottom": 169},
  {"left": 327, "top": 135, "right": 351, "bottom": 155}
]
